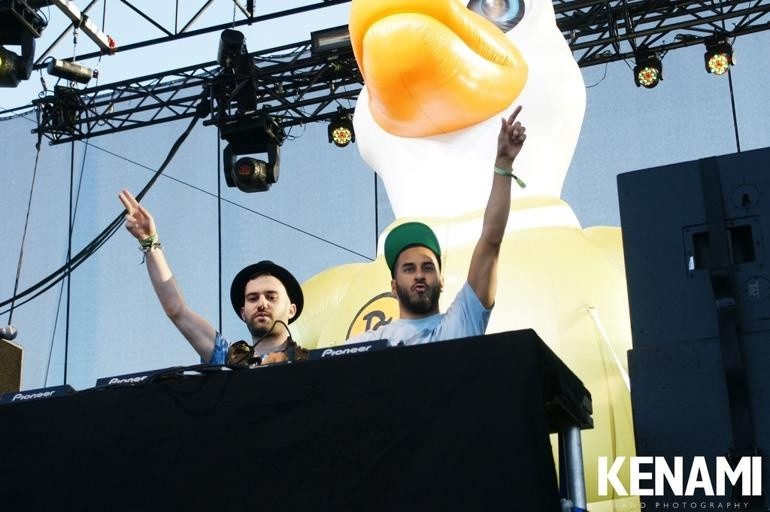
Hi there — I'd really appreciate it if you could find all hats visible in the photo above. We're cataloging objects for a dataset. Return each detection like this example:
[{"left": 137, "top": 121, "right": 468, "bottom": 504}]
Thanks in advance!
[
  {"left": 230, "top": 260, "right": 304, "bottom": 324},
  {"left": 384, "top": 222, "right": 441, "bottom": 270}
]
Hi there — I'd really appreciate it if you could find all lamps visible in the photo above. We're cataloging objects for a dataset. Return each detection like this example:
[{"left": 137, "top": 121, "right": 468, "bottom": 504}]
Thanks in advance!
[
  {"left": 327, "top": 114, "right": 356, "bottom": 146},
  {"left": 218, "top": 109, "right": 285, "bottom": 193},
  {"left": 633, "top": 38, "right": 734, "bottom": 89}
]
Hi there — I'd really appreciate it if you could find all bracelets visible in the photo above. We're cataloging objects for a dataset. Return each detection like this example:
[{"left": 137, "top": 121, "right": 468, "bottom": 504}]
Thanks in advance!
[
  {"left": 493, "top": 165, "right": 526, "bottom": 189},
  {"left": 137, "top": 233, "right": 165, "bottom": 265}
]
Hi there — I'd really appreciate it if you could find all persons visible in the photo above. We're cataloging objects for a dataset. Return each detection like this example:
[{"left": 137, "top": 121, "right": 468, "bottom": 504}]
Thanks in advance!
[
  {"left": 118, "top": 188, "right": 309, "bottom": 370},
  {"left": 338, "top": 104, "right": 526, "bottom": 348}
]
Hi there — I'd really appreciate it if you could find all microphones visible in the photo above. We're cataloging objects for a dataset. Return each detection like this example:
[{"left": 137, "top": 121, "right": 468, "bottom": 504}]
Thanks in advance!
[{"left": 0, "top": 325, "right": 18, "bottom": 341}]
